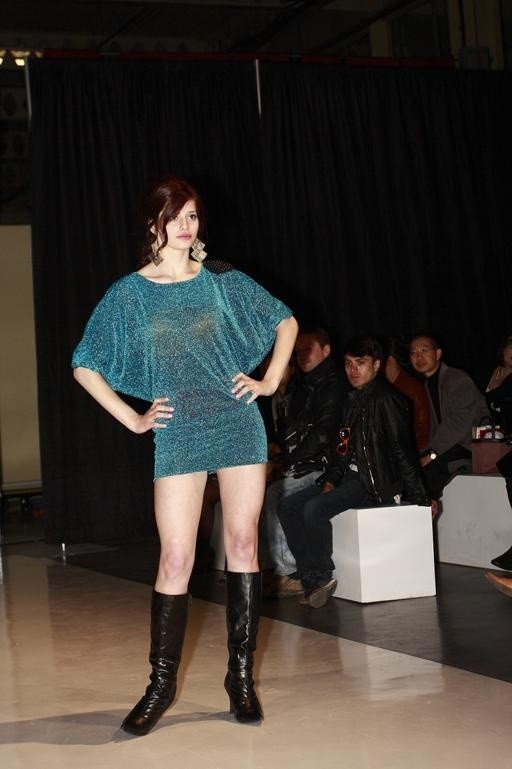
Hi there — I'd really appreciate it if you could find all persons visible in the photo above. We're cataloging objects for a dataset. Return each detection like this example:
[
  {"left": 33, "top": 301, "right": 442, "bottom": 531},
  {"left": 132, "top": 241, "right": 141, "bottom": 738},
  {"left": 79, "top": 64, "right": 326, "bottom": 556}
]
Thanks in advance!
[
  {"left": 70, "top": 177, "right": 300, "bottom": 737},
  {"left": 485, "top": 342, "right": 510, "bottom": 570},
  {"left": 210, "top": 322, "right": 442, "bottom": 606},
  {"left": 408, "top": 328, "right": 490, "bottom": 474}
]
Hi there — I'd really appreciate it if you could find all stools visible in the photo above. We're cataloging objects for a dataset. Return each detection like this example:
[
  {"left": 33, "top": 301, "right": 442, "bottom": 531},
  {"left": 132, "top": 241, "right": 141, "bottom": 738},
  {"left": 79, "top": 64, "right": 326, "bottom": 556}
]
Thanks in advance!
[
  {"left": 434, "top": 465, "right": 511, "bottom": 572},
  {"left": 328, "top": 500, "right": 439, "bottom": 607}
]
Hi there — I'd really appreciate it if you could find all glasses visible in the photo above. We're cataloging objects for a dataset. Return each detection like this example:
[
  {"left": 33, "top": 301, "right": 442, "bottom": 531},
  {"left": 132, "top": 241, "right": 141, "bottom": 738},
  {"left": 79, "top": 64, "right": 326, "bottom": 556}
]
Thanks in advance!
[{"left": 335, "top": 427, "right": 351, "bottom": 457}]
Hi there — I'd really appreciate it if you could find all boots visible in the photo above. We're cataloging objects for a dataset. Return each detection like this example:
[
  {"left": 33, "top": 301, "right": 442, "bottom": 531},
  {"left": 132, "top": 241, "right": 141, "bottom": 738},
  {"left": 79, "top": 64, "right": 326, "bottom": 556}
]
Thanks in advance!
[
  {"left": 119, "top": 588, "right": 190, "bottom": 737},
  {"left": 223, "top": 570, "right": 265, "bottom": 725}
]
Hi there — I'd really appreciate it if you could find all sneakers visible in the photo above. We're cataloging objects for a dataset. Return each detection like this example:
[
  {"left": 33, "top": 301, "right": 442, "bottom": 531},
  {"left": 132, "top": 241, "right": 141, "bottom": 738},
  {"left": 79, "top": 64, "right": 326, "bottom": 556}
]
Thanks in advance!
[
  {"left": 490, "top": 546, "right": 512, "bottom": 571},
  {"left": 484, "top": 571, "right": 511, "bottom": 599},
  {"left": 263, "top": 568, "right": 338, "bottom": 610}
]
